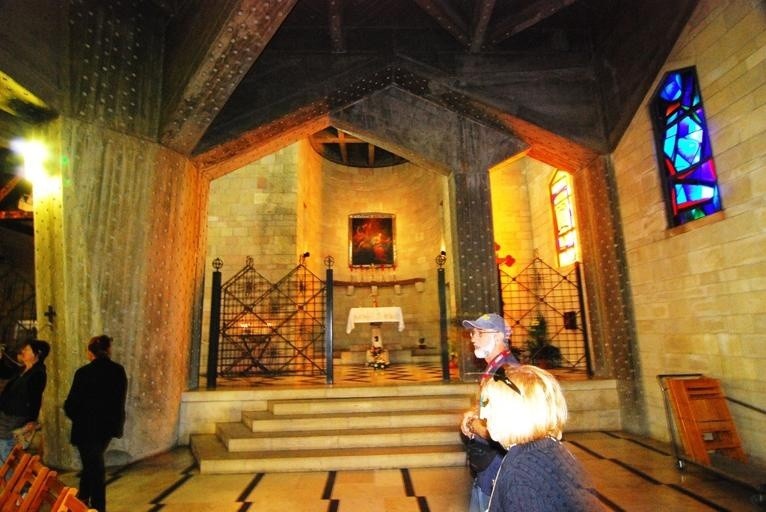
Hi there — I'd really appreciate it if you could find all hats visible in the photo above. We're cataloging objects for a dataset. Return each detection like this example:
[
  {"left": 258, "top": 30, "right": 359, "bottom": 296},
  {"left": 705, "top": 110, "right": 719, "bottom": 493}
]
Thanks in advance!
[
  {"left": 462, "top": 313, "right": 511, "bottom": 333},
  {"left": 29, "top": 340, "right": 51, "bottom": 362},
  {"left": 89, "top": 335, "right": 112, "bottom": 358}
]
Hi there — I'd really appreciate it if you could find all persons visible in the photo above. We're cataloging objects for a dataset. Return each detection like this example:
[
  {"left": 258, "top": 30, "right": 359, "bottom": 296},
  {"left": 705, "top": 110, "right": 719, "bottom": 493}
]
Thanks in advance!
[
  {"left": 60, "top": 334, "right": 128, "bottom": 511},
  {"left": 472, "top": 363, "right": 602, "bottom": 512},
  {"left": 460, "top": 312, "right": 521, "bottom": 512},
  {"left": 0, "top": 339, "right": 51, "bottom": 488}
]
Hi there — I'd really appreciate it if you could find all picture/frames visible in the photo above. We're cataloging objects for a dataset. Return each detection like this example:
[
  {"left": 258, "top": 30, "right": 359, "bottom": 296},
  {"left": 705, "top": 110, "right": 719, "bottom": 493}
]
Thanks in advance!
[{"left": 347, "top": 213, "right": 397, "bottom": 269}]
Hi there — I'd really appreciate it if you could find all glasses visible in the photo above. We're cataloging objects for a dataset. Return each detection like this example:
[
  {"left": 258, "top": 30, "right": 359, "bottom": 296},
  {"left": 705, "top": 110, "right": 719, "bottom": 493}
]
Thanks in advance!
[
  {"left": 469, "top": 330, "right": 490, "bottom": 337},
  {"left": 493, "top": 367, "right": 521, "bottom": 395}
]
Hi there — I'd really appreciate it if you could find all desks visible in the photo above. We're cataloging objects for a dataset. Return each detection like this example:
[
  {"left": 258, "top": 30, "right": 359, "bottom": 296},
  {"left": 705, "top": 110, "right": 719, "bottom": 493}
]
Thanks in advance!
[
  {"left": 346, "top": 306, "right": 403, "bottom": 365},
  {"left": 225, "top": 333, "right": 279, "bottom": 377}
]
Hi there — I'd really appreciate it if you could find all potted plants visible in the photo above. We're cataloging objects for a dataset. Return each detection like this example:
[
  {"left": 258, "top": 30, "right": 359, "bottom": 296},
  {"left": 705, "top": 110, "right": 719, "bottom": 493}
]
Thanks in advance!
[{"left": 528, "top": 316, "right": 560, "bottom": 370}]
